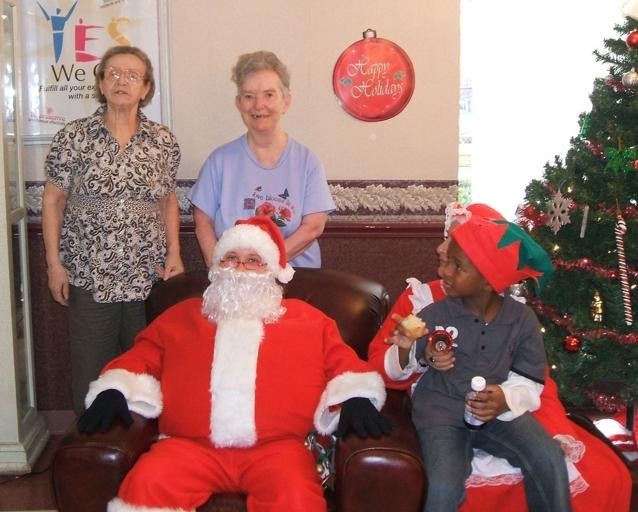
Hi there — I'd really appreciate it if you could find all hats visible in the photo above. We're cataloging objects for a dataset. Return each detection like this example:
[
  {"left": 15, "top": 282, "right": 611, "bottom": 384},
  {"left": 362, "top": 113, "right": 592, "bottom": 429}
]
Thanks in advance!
[
  {"left": 444, "top": 202, "right": 555, "bottom": 297},
  {"left": 212, "top": 216, "right": 295, "bottom": 285}
]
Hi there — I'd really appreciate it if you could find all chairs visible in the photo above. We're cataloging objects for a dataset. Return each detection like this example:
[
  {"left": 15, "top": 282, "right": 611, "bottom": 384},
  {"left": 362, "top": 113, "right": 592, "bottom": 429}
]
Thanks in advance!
[{"left": 51, "top": 268, "right": 428, "bottom": 512}]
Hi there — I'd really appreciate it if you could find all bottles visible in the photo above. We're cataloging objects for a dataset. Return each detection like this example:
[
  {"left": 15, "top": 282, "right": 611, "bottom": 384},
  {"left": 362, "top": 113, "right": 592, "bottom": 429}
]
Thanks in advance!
[{"left": 463, "top": 376, "right": 487, "bottom": 430}]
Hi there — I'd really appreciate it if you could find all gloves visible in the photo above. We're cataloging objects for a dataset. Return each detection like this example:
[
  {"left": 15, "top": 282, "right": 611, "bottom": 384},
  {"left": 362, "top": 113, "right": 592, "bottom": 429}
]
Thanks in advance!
[
  {"left": 77, "top": 390, "right": 134, "bottom": 434},
  {"left": 332, "top": 397, "right": 392, "bottom": 439}
]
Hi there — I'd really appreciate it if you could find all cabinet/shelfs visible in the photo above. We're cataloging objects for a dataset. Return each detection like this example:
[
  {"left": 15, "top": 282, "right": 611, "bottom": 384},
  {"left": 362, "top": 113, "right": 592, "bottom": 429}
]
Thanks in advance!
[{"left": 1, "top": 1, "right": 54, "bottom": 474}]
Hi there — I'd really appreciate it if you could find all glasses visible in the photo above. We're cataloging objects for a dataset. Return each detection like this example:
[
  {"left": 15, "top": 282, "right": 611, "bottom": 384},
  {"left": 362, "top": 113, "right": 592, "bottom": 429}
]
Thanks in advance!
[
  {"left": 221, "top": 255, "right": 267, "bottom": 270},
  {"left": 102, "top": 65, "right": 144, "bottom": 83}
]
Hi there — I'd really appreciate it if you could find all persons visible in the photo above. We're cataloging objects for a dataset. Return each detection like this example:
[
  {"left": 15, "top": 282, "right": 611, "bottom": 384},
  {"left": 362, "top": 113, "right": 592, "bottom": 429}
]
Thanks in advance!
[{"left": 84, "top": 205, "right": 386, "bottom": 512}]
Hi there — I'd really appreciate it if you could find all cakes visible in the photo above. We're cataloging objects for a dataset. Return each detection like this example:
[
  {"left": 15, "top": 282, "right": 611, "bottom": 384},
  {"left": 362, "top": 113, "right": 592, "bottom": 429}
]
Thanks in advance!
[{"left": 401, "top": 315, "right": 425, "bottom": 338}]
